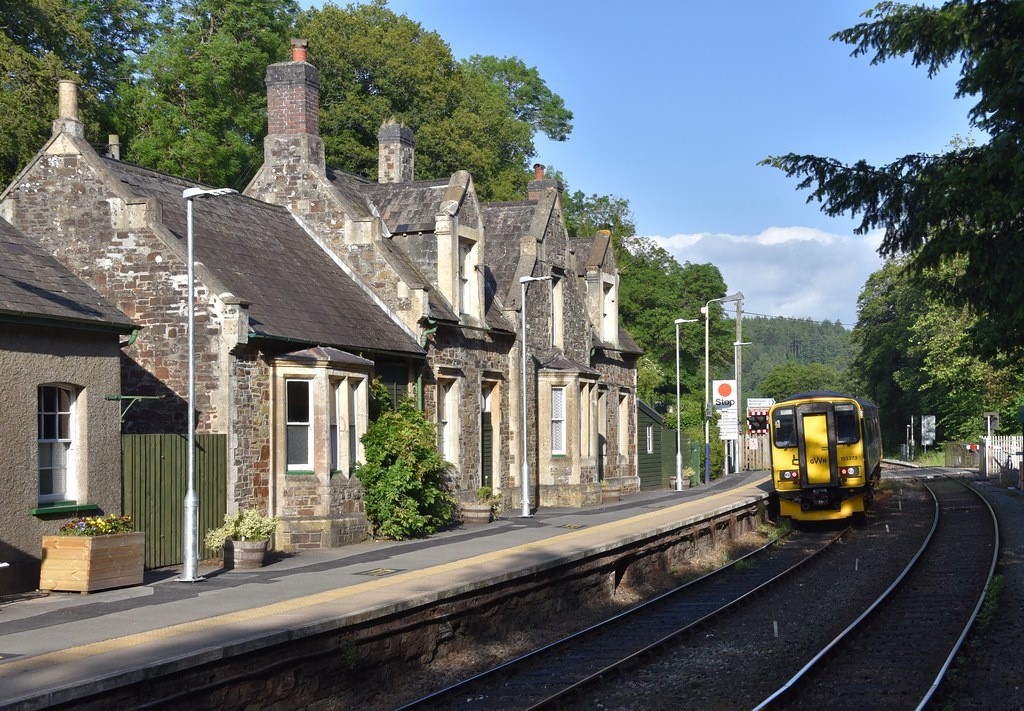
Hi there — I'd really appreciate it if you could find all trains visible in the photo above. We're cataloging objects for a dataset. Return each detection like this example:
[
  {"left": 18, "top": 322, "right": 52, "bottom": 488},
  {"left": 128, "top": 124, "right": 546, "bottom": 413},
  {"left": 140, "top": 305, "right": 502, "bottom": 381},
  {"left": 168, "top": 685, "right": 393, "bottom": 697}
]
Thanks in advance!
[{"left": 768, "top": 390, "right": 884, "bottom": 527}]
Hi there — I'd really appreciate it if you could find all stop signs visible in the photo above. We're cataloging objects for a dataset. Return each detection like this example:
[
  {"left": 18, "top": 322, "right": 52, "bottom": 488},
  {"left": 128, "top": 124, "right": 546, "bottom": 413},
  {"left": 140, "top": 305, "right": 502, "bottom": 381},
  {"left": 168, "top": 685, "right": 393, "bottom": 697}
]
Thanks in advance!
[{"left": 715, "top": 383, "right": 735, "bottom": 406}]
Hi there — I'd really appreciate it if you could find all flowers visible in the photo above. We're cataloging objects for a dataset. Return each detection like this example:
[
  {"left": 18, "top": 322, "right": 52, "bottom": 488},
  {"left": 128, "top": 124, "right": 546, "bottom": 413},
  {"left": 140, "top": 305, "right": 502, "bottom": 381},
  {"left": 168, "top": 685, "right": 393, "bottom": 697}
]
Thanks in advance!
[{"left": 62, "top": 514, "right": 133, "bottom": 535}]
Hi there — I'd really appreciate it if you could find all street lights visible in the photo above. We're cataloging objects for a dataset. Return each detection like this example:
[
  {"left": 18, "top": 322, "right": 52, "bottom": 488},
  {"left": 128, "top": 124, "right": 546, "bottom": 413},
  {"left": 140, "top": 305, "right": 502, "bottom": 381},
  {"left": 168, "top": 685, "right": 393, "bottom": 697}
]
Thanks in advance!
[
  {"left": 674, "top": 317, "right": 700, "bottom": 492},
  {"left": 520, "top": 275, "right": 554, "bottom": 517},
  {"left": 170, "top": 184, "right": 237, "bottom": 584}
]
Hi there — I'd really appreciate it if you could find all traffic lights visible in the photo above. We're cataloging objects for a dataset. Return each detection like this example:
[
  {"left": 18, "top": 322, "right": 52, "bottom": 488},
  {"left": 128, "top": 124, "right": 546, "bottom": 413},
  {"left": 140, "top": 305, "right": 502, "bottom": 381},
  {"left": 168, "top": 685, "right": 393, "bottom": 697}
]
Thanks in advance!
[{"left": 747, "top": 415, "right": 766, "bottom": 430}]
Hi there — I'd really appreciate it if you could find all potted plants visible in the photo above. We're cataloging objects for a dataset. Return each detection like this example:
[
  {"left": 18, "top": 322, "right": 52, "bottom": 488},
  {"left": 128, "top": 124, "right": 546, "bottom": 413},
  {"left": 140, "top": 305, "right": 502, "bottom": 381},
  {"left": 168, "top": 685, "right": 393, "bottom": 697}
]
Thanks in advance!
[
  {"left": 670, "top": 467, "right": 696, "bottom": 490},
  {"left": 204, "top": 510, "right": 281, "bottom": 569},
  {"left": 460, "top": 486, "right": 502, "bottom": 524}
]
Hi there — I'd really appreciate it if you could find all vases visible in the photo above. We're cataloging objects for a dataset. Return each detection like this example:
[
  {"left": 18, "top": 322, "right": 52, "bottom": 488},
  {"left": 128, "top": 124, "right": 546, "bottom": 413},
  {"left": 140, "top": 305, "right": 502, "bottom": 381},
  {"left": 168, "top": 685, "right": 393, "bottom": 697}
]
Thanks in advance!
[{"left": 39, "top": 531, "right": 146, "bottom": 596}]
